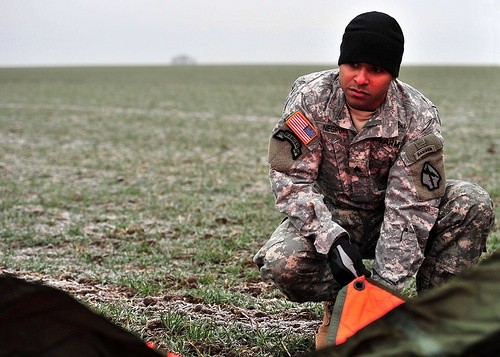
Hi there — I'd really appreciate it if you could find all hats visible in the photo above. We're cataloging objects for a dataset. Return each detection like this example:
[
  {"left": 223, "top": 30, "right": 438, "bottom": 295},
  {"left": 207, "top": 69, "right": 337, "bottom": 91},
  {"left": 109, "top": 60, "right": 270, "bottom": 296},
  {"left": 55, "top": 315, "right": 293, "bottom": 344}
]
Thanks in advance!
[{"left": 339, "top": 11, "right": 405, "bottom": 78}]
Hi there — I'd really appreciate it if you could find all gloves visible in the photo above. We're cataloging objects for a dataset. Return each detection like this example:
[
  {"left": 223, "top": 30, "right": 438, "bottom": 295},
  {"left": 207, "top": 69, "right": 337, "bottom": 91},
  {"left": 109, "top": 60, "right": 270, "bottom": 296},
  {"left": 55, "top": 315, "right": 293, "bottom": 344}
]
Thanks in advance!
[{"left": 326, "top": 240, "right": 372, "bottom": 287}]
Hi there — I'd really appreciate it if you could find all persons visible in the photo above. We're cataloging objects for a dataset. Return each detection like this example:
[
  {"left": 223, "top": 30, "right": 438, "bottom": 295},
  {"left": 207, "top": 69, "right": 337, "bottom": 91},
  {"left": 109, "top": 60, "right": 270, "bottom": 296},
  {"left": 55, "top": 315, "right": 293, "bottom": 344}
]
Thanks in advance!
[{"left": 252, "top": 11, "right": 495, "bottom": 352}]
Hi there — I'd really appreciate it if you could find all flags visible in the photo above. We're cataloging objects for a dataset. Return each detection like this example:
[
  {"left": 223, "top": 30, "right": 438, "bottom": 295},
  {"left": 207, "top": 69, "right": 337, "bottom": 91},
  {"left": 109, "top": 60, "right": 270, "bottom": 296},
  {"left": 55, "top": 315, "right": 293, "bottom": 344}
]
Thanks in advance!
[{"left": 285, "top": 112, "right": 319, "bottom": 146}]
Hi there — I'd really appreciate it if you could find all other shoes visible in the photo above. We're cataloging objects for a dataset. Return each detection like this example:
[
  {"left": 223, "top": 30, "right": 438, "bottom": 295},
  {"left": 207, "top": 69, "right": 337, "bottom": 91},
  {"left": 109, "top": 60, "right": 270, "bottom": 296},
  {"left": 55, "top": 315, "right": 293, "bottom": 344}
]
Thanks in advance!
[{"left": 314, "top": 301, "right": 331, "bottom": 349}]
